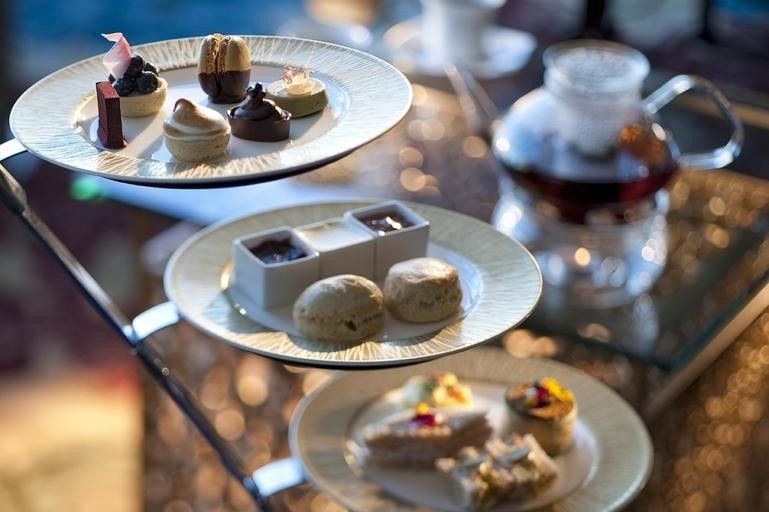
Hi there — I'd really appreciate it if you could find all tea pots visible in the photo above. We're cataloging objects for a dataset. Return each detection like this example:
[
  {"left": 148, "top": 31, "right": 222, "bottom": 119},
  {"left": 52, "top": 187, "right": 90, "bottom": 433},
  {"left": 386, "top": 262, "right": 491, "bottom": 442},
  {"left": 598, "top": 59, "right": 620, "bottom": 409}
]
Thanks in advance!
[
  {"left": 488, "top": 190, "right": 672, "bottom": 311},
  {"left": 441, "top": 41, "right": 746, "bottom": 227}
]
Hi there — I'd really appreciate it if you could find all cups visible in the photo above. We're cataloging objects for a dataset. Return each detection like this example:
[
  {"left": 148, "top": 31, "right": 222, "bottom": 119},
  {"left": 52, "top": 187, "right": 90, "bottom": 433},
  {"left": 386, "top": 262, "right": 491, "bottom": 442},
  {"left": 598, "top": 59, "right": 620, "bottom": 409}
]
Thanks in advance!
[{"left": 417, "top": 1, "right": 506, "bottom": 60}]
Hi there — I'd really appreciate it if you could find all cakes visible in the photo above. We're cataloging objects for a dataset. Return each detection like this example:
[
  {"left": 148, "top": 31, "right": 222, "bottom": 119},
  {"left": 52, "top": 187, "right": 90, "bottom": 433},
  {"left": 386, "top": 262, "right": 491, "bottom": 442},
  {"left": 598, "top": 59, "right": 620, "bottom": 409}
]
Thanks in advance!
[
  {"left": 358, "top": 372, "right": 580, "bottom": 512},
  {"left": 290, "top": 272, "right": 388, "bottom": 344},
  {"left": 383, "top": 256, "right": 464, "bottom": 323},
  {"left": 94, "top": 28, "right": 330, "bottom": 161}
]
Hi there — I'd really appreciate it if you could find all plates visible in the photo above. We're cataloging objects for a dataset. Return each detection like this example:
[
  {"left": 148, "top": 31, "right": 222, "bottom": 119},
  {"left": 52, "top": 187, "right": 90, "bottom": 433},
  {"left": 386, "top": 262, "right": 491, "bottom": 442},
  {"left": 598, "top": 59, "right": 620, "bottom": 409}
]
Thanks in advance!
[
  {"left": 160, "top": 197, "right": 542, "bottom": 369},
  {"left": 8, "top": 33, "right": 416, "bottom": 186},
  {"left": 389, "top": 19, "right": 540, "bottom": 83},
  {"left": 287, "top": 349, "right": 656, "bottom": 511}
]
